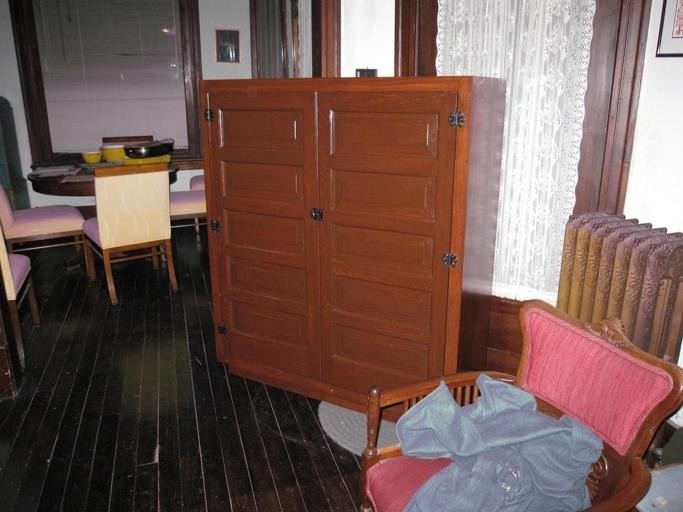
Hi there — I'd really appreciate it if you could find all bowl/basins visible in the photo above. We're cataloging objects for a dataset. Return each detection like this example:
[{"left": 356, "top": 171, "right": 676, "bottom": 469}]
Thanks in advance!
[{"left": 81, "top": 144, "right": 126, "bottom": 163}]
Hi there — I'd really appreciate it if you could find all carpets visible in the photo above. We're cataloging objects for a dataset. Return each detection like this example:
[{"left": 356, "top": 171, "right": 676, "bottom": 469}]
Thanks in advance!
[{"left": 317, "top": 396, "right": 403, "bottom": 459}]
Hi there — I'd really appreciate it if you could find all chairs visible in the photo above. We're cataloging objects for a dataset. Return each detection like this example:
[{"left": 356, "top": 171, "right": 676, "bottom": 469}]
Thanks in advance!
[
  {"left": 83, "top": 162, "right": 207, "bottom": 305},
  {"left": 356, "top": 299, "right": 683, "bottom": 512},
  {"left": 1, "top": 181, "right": 85, "bottom": 369}
]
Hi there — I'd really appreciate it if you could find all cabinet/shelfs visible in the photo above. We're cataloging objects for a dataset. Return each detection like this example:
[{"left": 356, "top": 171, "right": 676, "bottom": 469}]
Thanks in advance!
[{"left": 196, "top": 75, "right": 472, "bottom": 426}]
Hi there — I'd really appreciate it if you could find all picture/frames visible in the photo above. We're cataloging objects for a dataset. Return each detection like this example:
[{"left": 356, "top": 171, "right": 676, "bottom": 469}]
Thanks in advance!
[{"left": 654, "top": 0, "right": 682, "bottom": 57}]
